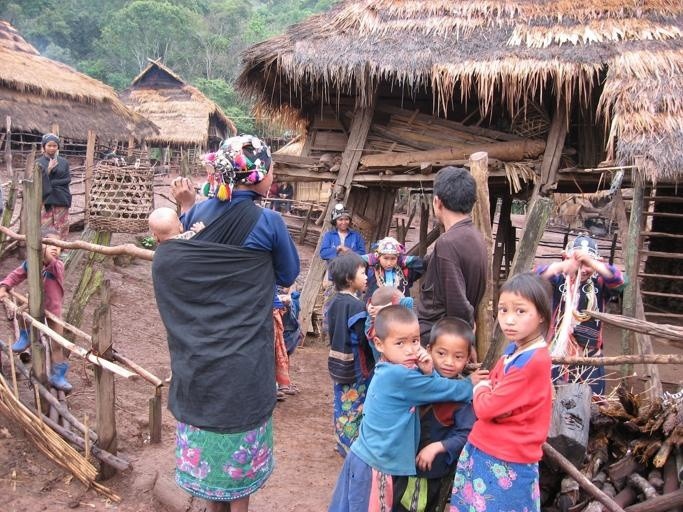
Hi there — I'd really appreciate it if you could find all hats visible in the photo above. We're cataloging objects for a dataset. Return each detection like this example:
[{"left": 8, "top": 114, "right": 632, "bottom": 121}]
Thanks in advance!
[
  {"left": 376, "top": 237, "right": 402, "bottom": 254},
  {"left": 330, "top": 204, "right": 352, "bottom": 222},
  {"left": 204, "top": 133, "right": 272, "bottom": 183},
  {"left": 42, "top": 132, "right": 59, "bottom": 147},
  {"left": 566, "top": 233, "right": 601, "bottom": 258}
]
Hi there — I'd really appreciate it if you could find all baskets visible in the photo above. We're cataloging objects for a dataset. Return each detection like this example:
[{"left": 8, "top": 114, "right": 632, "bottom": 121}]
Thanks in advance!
[{"left": 84, "top": 159, "right": 156, "bottom": 234}]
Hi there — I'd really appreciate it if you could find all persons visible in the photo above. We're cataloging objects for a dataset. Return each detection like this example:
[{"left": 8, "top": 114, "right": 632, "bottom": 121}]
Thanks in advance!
[
  {"left": 144, "top": 204, "right": 211, "bottom": 256},
  {"left": 273, "top": 284, "right": 303, "bottom": 398},
  {"left": 0, "top": 224, "right": 74, "bottom": 394},
  {"left": 417, "top": 167, "right": 487, "bottom": 365},
  {"left": 150, "top": 133, "right": 302, "bottom": 511},
  {"left": 361, "top": 236, "right": 424, "bottom": 300},
  {"left": 268, "top": 175, "right": 293, "bottom": 215},
  {"left": 328, "top": 306, "right": 490, "bottom": 512},
  {"left": 532, "top": 236, "right": 629, "bottom": 396},
  {"left": 31, "top": 131, "right": 72, "bottom": 253},
  {"left": 326, "top": 254, "right": 415, "bottom": 457},
  {"left": 450, "top": 272, "right": 553, "bottom": 512},
  {"left": 320, "top": 204, "right": 364, "bottom": 331},
  {"left": 393, "top": 317, "right": 477, "bottom": 512}
]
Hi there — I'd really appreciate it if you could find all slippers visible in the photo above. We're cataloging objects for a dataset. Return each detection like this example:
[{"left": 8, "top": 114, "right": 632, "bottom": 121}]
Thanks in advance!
[{"left": 273, "top": 383, "right": 295, "bottom": 400}]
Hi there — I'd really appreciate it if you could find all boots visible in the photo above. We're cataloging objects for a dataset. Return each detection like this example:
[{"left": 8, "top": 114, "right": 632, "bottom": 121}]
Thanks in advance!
[
  {"left": 50, "top": 361, "right": 72, "bottom": 390},
  {"left": 12, "top": 325, "right": 31, "bottom": 353}
]
